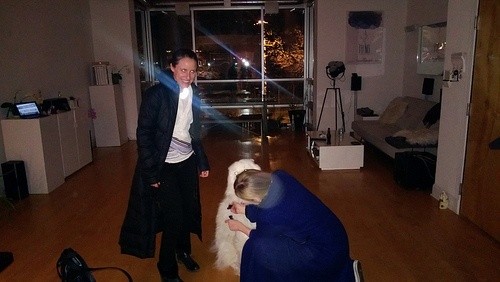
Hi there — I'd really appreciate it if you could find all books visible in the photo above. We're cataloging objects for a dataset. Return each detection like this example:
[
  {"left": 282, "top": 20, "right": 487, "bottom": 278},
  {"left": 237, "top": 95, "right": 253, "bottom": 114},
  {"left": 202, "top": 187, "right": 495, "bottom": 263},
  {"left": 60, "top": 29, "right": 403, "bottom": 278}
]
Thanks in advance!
[{"left": 92, "top": 63, "right": 108, "bottom": 86}]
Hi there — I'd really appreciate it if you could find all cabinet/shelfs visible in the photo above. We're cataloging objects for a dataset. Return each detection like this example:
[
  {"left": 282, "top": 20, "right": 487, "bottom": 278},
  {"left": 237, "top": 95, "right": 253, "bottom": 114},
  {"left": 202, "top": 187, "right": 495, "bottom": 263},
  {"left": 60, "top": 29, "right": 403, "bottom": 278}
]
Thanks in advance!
[
  {"left": 88, "top": 84, "right": 128, "bottom": 147},
  {"left": 1, "top": 107, "right": 94, "bottom": 194}
]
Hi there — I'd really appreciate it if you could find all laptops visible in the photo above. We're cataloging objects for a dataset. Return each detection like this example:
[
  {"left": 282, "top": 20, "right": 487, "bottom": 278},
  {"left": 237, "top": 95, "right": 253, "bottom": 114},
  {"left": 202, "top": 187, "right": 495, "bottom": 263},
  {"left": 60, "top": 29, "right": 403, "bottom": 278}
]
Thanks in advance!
[{"left": 14, "top": 101, "right": 50, "bottom": 119}]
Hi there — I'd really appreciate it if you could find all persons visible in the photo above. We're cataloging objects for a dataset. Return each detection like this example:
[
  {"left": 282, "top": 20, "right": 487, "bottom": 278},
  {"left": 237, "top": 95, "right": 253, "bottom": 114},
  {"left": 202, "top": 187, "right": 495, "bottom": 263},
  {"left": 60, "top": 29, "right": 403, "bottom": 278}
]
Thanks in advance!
[
  {"left": 225, "top": 169, "right": 365, "bottom": 282},
  {"left": 118, "top": 49, "right": 211, "bottom": 282}
]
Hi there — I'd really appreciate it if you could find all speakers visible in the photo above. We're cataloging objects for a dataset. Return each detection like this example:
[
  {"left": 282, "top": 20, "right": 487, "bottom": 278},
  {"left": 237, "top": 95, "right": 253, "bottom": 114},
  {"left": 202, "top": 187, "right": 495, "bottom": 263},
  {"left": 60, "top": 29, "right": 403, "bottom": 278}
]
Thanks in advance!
[{"left": 1, "top": 160, "right": 29, "bottom": 201}]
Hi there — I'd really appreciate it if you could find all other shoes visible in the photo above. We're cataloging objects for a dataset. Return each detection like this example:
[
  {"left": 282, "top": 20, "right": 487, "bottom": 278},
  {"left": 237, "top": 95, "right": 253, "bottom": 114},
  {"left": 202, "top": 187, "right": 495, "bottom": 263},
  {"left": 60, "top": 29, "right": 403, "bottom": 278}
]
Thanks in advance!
[
  {"left": 177, "top": 253, "right": 200, "bottom": 271},
  {"left": 157, "top": 262, "right": 185, "bottom": 282},
  {"left": 351, "top": 260, "right": 366, "bottom": 282}
]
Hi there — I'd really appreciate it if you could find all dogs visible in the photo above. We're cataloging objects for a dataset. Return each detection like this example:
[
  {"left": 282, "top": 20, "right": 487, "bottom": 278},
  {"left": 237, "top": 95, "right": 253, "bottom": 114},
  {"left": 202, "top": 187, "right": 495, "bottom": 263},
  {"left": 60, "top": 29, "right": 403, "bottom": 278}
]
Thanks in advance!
[{"left": 208, "top": 159, "right": 262, "bottom": 276}]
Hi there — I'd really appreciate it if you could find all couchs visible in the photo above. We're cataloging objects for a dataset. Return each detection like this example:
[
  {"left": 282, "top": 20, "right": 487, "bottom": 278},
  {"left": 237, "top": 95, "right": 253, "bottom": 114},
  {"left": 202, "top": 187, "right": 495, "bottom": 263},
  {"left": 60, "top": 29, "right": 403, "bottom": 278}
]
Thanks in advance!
[{"left": 351, "top": 96, "right": 441, "bottom": 172}]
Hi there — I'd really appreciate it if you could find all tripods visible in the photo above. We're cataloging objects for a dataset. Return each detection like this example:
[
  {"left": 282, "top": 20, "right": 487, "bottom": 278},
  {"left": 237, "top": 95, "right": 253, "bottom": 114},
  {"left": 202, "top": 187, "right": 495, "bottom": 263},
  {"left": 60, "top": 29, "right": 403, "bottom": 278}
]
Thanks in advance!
[{"left": 317, "top": 75, "right": 345, "bottom": 133}]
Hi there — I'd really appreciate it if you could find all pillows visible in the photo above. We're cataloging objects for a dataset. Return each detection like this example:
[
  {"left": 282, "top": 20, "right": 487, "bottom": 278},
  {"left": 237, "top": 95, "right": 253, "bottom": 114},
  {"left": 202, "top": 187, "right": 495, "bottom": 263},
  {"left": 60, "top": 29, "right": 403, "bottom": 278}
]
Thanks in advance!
[{"left": 382, "top": 103, "right": 408, "bottom": 125}]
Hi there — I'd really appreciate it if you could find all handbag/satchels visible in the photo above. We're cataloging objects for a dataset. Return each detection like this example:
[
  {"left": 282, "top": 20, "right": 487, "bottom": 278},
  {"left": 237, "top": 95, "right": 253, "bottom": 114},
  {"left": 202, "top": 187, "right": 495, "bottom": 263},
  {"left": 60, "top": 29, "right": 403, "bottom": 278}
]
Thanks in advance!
[
  {"left": 56, "top": 248, "right": 133, "bottom": 282},
  {"left": 393, "top": 146, "right": 437, "bottom": 189}
]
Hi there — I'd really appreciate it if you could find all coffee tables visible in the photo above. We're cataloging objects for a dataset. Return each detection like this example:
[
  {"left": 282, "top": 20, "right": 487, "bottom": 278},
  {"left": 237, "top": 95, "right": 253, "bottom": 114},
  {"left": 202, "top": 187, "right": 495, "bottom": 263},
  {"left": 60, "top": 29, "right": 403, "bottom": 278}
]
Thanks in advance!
[{"left": 305, "top": 129, "right": 364, "bottom": 171}]
